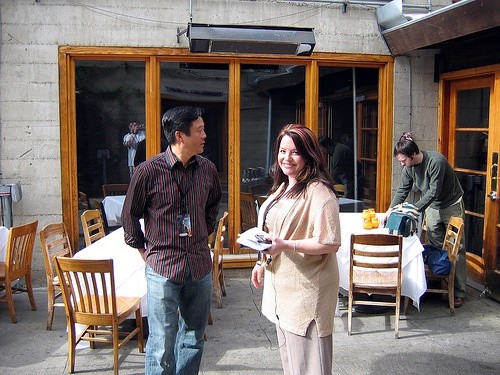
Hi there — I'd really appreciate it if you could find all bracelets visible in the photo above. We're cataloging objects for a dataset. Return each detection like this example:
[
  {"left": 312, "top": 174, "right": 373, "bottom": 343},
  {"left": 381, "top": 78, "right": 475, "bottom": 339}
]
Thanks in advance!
[{"left": 294, "top": 242, "right": 296, "bottom": 252}]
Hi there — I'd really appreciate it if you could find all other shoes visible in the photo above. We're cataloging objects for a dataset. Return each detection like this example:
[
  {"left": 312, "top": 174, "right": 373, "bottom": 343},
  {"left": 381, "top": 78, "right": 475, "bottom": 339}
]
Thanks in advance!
[{"left": 454, "top": 297, "right": 463, "bottom": 307}]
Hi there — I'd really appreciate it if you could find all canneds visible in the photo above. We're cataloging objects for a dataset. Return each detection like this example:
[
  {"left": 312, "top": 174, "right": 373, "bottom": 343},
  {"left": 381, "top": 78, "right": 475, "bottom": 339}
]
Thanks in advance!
[{"left": 362, "top": 208, "right": 379, "bottom": 229}]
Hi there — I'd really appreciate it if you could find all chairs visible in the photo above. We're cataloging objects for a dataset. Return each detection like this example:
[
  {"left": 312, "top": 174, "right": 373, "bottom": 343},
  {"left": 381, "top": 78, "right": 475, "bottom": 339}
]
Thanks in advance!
[
  {"left": 52, "top": 254, "right": 144, "bottom": 375},
  {"left": 77, "top": 191, "right": 99, "bottom": 234},
  {"left": 347, "top": 233, "right": 403, "bottom": 338},
  {"left": 0, "top": 220, "right": 38, "bottom": 323},
  {"left": 39, "top": 221, "right": 71, "bottom": 331},
  {"left": 211, "top": 211, "right": 229, "bottom": 310},
  {"left": 80, "top": 209, "right": 106, "bottom": 246},
  {"left": 102, "top": 183, "right": 129, "bottom": 196},
  {"left": 240, "top": 192, "right": 258, "bottom": 231}
]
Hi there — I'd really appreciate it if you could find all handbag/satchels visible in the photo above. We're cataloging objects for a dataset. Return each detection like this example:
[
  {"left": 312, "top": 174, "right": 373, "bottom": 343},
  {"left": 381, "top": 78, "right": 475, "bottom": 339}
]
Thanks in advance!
[{"left": 386, "top": 212, "right": 415, "bottom": 237}]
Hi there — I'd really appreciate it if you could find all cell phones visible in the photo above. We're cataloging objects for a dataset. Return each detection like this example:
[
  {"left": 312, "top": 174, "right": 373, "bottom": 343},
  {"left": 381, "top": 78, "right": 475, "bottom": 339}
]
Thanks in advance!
[{"left": 256, "top": 235, "right": 272, "bottom": 244}]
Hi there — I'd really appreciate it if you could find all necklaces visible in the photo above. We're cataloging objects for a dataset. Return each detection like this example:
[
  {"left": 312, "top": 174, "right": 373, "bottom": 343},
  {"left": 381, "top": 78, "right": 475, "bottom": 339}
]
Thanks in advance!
[{"left": 262, "top": 192, "right": 287, "bottom": 231}]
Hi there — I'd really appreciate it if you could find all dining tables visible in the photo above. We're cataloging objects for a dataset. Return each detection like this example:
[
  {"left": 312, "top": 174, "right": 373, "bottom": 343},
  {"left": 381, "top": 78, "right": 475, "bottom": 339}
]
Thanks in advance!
[{"left": 402, "top": 215, "right": 464, "bottom": 316}]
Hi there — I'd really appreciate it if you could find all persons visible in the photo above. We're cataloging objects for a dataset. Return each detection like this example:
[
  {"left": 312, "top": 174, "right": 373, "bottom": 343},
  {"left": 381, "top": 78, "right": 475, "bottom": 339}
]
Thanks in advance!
[
  {"left": 123, "top": 121, "right": 145, "bottom": 177},
  {"left": 318, "top": 135, "right": 364, "bottom": 200},
  {"left": 121, "top": 104, "right": 222, "bottom": 375},
  {"left": 380, "top": 131, "right": 466, "bottom": 308},
  {"left": 251, "top": 124, "right": 342, "bottom": 375}
]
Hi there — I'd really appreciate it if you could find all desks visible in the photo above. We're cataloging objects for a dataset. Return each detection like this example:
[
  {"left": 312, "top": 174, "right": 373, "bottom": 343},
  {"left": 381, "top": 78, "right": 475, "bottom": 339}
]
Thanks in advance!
[
  {"left": 103, "top": 196, "right": 125, "bottom": 228},
  {"left": 67, "top": 218, "right": 149, "bottom": 339},
  {"left": 339, "top": 197, "right": 363, "bottom": 212},
  {"left": 336, "top": 211, "right": 427, "bottom": 314}
]
